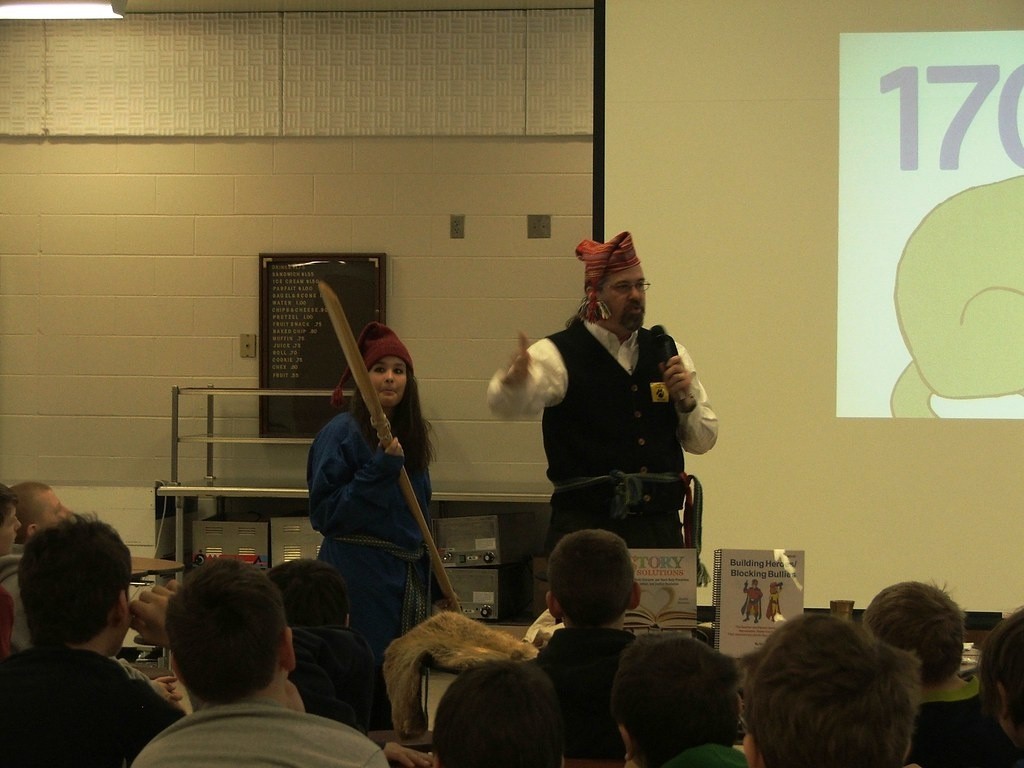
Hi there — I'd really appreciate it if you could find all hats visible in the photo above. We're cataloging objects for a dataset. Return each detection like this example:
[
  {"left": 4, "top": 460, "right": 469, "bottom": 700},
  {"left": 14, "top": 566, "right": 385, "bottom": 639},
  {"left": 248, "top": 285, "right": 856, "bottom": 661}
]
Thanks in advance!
[
  {"left": 330, "top": 321, "right": 415, "bottom": 410},
  {"left": 577, "top": 231, "right": 641, "bottom": 323}
]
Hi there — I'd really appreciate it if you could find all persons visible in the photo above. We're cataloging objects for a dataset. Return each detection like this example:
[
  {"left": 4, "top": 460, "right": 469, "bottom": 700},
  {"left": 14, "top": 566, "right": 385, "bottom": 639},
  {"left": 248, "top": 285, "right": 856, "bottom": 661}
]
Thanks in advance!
[
  {"left": 0, "top": 479, "right": 1024, "bottom": 768},
  {"left": 305, "top": 322, "right": 459, "bottom": 676},
  {"left": 487, "top": 231, "right": 717, "bottom": 551}
]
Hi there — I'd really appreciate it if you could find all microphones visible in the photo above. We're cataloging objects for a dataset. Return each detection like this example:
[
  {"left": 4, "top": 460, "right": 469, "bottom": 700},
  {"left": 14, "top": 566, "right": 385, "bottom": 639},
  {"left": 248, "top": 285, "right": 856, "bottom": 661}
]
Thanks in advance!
[{"left": 651, "top": 325, "right": 687, "bottom": 400}]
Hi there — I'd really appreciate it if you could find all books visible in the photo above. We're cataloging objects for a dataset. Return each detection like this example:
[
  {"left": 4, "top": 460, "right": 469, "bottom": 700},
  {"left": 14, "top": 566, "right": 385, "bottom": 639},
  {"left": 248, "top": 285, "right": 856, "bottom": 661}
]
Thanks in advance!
[
  {"left": 622, "top": 549, "right": 697, "bottom": 640},
  {"left": 710, "top": 549, "right": 806, "bottom": 658}
]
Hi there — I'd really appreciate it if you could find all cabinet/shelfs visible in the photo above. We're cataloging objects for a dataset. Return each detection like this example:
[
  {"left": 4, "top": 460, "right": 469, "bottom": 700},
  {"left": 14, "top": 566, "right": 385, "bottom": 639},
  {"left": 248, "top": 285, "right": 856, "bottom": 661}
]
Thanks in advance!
[{"left": 171, "top": 384, "right": 355, "bottom": 485}]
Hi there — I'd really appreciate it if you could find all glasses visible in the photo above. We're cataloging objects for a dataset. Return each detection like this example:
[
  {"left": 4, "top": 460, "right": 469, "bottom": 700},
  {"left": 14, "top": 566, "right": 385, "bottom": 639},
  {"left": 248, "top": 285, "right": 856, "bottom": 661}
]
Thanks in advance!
[{"left": 615, "top": 281, "right": 651, "bottom": 293}]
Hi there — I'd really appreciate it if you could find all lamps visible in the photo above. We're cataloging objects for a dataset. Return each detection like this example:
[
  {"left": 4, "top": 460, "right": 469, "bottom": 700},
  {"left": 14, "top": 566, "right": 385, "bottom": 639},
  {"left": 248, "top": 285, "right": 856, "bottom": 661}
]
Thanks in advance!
[{"left": 0, "top": 0, "right": 127, "bottom": 19}]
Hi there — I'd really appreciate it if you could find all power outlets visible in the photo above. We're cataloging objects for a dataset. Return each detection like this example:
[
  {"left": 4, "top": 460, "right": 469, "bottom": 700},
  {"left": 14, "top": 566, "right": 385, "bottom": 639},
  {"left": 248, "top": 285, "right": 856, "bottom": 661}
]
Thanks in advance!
[{"left": 450, "top": 213, "right": 465, "bottom": 239}]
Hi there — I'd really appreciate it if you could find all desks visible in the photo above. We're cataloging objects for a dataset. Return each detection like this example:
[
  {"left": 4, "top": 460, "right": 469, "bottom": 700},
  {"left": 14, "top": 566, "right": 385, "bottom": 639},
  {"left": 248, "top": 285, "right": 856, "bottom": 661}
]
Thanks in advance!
[
  {"left": 157, "top": 486, "right": 553, "bottom": 674},
  {"left": 122, "top": 557, "right": 186, "bottom": 676}
]
